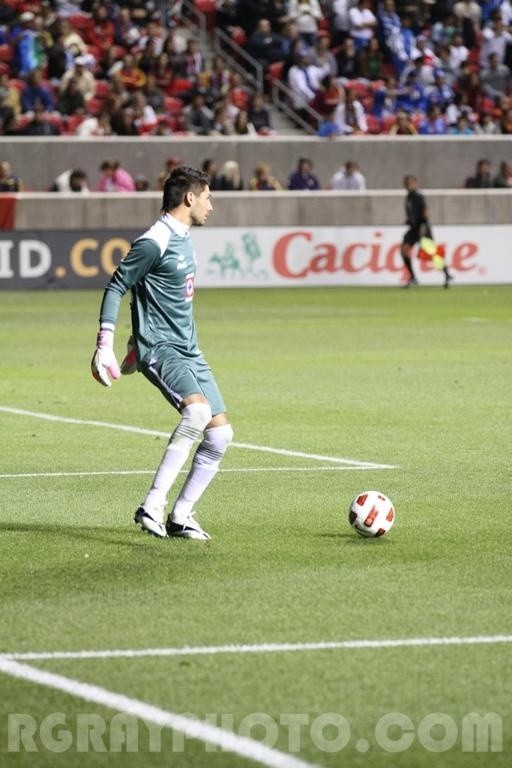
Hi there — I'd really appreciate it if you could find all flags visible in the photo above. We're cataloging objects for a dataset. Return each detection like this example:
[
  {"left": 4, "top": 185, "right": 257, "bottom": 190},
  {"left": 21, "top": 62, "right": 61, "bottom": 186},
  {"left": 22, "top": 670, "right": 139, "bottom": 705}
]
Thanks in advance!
[{"left": 417, "top": 235, "right": 448, "bottom": 271}]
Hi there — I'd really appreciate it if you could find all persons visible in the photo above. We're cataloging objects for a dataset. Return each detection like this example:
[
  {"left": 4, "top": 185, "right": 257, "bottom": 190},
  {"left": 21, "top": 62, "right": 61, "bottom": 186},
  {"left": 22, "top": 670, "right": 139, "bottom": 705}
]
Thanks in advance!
[
  {"left": 490, "top": 159, "right": 512, "bottom": 187},
  {"left": 322, "top": 160, "right": 367, "bottom": 190},
  {"left": 285, "top": 155, "right": 320, "bottom": 190},
  {"left": 215, "top": 158, "right": 245, "bottom": 189},
  {"left": 248, "top": 162, "right": 283, "bottom": 191},
  {"left": 132, "top": 173, "right": 151, "bottom": 191},
  {"left": 199, "top": 157, "right": 219, "bottom": 190},
  {"left": 399, "top": 172, "right": 454, "bottom": 290},
  {"left": 0, "top": 0, "right": 512, "bottom": 140},
  {"left": 45, "top": 166, "right": 90, "bottom": 192},
  {"left": 90, "top": 162, "right": 237, "bottom": 542},
  {"left": 156, "top": 154, "right": 182, "bottom": 191},
  {"left": 464, "top": 157, "right": 496, "bottom": 187},
  {"left": 0, "top": 158, "right": 23, "bottom": 192},
  {"left": 95, "top": 157, "right": 138, "bottom": 192}
]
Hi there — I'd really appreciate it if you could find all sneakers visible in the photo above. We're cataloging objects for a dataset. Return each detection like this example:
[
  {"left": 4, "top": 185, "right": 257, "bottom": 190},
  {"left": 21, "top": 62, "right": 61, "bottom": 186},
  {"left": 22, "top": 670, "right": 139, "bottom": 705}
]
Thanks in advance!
[
  {"left": 165, "top": 513, "right": 210, "bottom": 540},
  {"left": 404, "top": 276, "right": 453, "bottom": 288},
  {"left": 134, "top": 506, "right": 169, "bottom": 538}
]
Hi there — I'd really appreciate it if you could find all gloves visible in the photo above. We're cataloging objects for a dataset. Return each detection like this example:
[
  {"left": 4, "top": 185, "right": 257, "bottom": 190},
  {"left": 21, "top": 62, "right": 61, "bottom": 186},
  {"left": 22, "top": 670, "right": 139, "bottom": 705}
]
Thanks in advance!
[
  {"left": 121, "top": 337, "right": 138, "bottom": 375},
  {"left": 91, "top": 322, "right": 121, "bottom": 387}
]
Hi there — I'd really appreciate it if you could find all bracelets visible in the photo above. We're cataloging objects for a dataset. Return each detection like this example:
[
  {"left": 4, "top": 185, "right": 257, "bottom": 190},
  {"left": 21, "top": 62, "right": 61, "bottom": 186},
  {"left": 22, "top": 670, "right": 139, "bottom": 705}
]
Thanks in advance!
[{"left": 98, "top": 326, "right": 114, "bottom": 334}]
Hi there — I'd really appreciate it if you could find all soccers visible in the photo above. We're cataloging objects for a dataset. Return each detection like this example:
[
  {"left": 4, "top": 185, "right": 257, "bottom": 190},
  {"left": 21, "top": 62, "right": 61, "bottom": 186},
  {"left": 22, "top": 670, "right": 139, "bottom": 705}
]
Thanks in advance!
[{"left": 348, "top": 489, "right": 395, "bottom": 536}]
